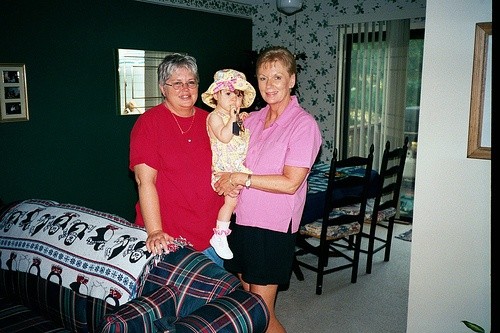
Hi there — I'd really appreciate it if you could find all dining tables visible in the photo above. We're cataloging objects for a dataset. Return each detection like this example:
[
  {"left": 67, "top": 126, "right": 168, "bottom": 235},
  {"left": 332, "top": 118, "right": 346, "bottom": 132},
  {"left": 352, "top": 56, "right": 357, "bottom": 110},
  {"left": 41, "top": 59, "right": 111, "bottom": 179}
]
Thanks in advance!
[{"left": 301, "top": 161, "right": 378, "bottom": 227}]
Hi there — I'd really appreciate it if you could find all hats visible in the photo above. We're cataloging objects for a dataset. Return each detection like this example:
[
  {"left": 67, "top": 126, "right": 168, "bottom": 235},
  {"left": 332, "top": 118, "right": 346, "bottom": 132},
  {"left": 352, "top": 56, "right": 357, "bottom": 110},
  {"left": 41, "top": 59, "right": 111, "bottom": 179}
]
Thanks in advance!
[{"left": 201, "top": 69, "right": 256, "bottom": 109}]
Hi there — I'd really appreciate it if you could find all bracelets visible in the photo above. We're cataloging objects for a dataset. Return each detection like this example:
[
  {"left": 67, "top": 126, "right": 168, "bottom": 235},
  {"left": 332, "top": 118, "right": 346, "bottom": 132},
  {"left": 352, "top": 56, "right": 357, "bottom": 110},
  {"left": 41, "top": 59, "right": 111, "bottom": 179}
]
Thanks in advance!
[{"left": 229, "top": 173, "right": 234, "bottom": 186}]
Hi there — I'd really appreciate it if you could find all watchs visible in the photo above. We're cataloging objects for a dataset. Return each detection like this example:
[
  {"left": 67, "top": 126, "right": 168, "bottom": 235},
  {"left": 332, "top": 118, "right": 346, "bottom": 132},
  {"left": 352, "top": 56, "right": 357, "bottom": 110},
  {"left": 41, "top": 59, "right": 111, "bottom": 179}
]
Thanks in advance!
[{"left": 246, "top": 174, "right": 251, "bottom": 187}]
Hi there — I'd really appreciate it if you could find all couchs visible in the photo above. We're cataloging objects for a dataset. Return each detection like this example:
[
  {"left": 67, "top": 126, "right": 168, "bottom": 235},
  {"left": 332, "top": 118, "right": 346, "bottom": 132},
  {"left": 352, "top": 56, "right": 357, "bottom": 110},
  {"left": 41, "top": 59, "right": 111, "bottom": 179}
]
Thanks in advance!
[{"left": 0, "top": 199, "right": 270, "bottom": 333}]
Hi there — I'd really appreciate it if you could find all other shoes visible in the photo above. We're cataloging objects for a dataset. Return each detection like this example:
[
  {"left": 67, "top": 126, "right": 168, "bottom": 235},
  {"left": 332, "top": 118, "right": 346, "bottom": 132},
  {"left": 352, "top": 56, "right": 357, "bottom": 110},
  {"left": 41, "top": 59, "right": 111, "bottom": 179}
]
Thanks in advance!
[{"left": 209, "top": 228, "right": 234, "bottom": 259}]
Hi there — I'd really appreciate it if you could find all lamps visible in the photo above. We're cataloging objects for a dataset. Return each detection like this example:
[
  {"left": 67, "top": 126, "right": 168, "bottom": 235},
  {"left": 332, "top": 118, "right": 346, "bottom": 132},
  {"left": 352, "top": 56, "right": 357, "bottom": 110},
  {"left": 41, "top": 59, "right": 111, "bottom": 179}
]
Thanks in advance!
[{"left": 277, "top": 0, "right": 303, "bottom": 15}]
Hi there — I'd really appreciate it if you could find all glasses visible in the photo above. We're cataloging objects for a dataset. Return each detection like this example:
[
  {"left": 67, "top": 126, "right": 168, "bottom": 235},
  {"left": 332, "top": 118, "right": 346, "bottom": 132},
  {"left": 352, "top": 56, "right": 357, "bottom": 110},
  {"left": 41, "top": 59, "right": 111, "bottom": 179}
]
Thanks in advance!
[{"left": 164, "top": 79, "right": 199, "bottom": 90}]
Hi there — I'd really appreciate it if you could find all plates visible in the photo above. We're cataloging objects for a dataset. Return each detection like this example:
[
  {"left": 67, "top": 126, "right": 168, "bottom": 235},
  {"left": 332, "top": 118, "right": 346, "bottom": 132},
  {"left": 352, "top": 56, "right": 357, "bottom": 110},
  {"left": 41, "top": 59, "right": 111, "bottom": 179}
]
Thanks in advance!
[{"left": 324, "top": 170, "right": 347, "bottom": 179}]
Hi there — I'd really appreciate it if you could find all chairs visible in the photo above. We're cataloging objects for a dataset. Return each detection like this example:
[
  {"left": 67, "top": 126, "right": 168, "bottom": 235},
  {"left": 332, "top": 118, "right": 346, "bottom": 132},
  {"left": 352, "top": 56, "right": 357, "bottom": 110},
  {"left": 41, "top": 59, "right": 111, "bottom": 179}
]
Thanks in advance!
[{"left": 293, "top": 139, "right": 408, "bottom": 295}]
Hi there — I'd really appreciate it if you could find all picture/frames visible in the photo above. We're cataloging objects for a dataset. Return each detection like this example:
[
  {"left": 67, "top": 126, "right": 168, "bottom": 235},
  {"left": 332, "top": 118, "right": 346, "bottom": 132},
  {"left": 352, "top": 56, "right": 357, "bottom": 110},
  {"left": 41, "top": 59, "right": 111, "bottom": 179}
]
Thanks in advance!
[
  {"left": 0, "top": 63, "right": 30, "bottom": 122},
  {"left": 467, "top": 22, "right": 491, "bottom": 160}
]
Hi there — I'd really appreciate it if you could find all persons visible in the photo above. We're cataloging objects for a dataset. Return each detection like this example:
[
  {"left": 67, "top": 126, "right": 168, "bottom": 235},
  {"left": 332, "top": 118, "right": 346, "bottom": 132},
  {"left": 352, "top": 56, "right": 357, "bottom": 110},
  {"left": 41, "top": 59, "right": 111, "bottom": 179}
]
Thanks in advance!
[
  {"left": 215, "top": 47, "right": 322, "bottom": 333},
  {"left": 129, "top": 54, "right": 223, "bottom": 269},
  {"left": 201, "top": 69, "right": 256, "bottom": 259}
]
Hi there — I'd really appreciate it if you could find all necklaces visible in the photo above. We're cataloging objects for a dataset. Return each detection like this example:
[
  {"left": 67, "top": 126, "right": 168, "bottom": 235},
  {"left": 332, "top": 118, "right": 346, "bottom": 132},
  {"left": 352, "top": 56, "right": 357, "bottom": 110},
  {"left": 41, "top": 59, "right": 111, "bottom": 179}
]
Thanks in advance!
[{"left": 171, "top": 113, "right": 195, "bottom": 142}]
